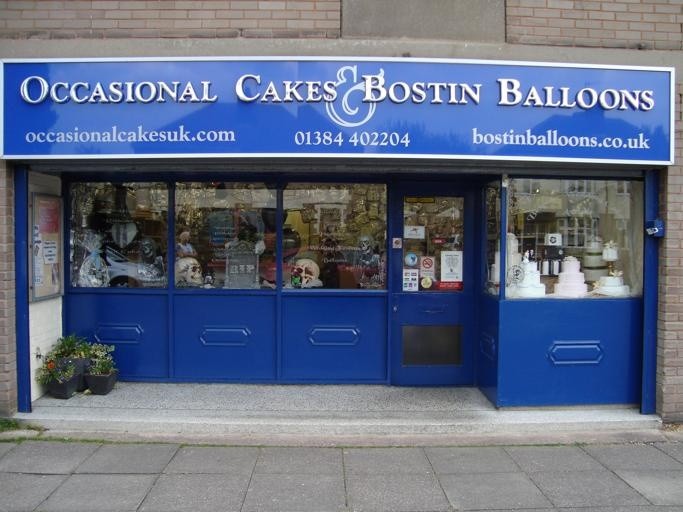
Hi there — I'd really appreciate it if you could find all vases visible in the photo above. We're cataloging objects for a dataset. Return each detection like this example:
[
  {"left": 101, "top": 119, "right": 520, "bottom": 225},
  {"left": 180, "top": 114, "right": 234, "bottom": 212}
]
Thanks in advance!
[{"left": 48, "top": 376, "right": 76, "bottom": 400}]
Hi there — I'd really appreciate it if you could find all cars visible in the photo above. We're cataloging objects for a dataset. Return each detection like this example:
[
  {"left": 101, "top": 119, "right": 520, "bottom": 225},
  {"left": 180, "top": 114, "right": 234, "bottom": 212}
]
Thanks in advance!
[
  {"left": 69, "top": 238, "right": 167, "bottom": 288},
  {"left": 266, "top": 246, "right": 438, "bottom": 291}
]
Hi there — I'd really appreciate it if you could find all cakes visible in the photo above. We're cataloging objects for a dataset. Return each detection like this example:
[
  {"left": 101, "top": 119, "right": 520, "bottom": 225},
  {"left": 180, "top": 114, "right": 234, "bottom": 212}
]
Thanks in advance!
[
  {"left": 582, "top": 236, "right": 611, "bottom": 283},
  {"left": 494, "top": 232, "right": 524, "bottom": 283},
  {"left": 554, "top": 255, "right": 588, "bottom": 294},
  {"left": 506, "top": 250, "right": 546, "bottom": 297},
  {"left": 596, "top": 241, "right": 631, "bottom": 298}
]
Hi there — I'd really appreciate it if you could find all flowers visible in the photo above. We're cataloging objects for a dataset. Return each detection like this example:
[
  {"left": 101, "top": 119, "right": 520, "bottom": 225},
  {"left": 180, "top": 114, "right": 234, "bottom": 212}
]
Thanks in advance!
[{"left": 35, "top": 350, "right": 76, "bottom": 387}]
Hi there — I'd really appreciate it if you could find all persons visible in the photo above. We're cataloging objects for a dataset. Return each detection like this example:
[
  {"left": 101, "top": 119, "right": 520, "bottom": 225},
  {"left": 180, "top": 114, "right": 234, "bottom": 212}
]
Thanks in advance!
[{"left": 175, "top": 231, "right": 198, "bottom": 257}]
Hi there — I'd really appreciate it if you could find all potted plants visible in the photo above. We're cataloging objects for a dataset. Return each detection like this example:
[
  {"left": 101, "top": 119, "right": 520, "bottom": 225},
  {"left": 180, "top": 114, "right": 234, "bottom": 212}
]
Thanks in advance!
[{"left": 49, "top": 332, "right": 118, "bottom": 395}]
